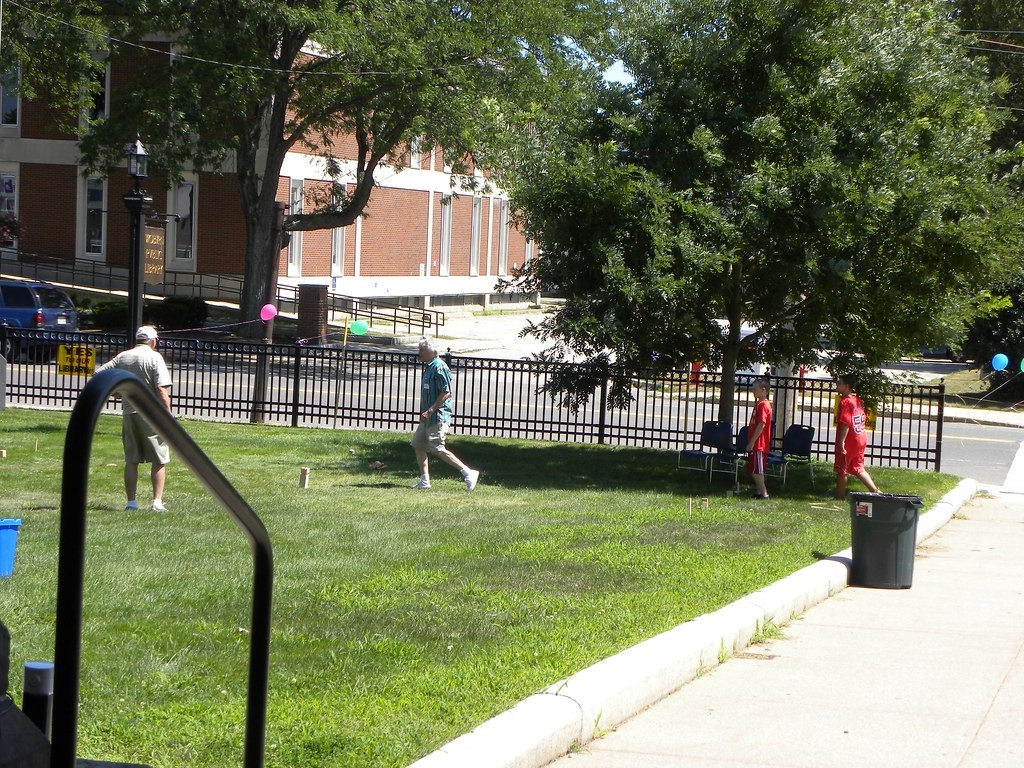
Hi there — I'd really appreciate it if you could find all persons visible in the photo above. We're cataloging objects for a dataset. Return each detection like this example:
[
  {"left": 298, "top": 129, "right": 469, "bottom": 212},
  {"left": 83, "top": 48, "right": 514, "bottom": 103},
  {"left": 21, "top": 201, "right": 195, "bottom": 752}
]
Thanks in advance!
[
  {"left": 93, "top": 324, "right": 174, "bottom": 513},
  {"left": 746, "top": 378, "right": 773, "bottom": 499},
  {"left": 409, "top": 336, "right": 480, "bottom": 492},
  {"left": 833, "top": 374, "right": 884, "bottom": 496}
]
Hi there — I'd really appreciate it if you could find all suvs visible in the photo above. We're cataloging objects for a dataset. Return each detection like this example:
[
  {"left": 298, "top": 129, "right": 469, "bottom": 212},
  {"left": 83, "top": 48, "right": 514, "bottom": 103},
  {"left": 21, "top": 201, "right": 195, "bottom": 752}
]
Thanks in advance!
[{"left": 0, "top": 278, "right": 79, "bottom": 365}]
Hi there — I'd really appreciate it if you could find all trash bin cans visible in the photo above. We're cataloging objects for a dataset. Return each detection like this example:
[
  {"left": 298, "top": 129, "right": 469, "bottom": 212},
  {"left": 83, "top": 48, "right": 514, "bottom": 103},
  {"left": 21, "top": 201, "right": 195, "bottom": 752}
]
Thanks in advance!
[{"left": 848, "top": 492, "right": 925, "bottom": 590}]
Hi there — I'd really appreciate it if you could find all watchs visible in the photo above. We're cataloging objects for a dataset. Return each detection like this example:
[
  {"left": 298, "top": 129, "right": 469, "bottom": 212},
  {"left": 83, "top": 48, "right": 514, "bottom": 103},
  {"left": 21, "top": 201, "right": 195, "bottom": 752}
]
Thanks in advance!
[{"left": 428, "top": 408, "right": 435, "bottom": 415}]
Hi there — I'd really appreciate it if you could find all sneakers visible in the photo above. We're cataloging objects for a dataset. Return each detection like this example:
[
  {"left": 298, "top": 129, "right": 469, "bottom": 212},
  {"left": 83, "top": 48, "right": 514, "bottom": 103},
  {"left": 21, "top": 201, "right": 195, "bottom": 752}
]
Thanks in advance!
[
  {"left": 464, "top": 470, "right": 479, "bottom": 491},
  {"left": 151, "top": 504, "right": 169, "bottom": 512},
  {"left": 413, "top": 484, "right": 432, "bottom": 488}
]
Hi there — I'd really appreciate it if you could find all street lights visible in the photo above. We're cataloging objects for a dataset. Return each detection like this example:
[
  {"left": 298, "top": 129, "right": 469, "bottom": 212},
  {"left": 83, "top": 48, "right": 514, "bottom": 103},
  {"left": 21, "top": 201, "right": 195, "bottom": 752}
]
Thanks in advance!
[{"left": 123, "top": 130, "right": 154, "bottom": 352}]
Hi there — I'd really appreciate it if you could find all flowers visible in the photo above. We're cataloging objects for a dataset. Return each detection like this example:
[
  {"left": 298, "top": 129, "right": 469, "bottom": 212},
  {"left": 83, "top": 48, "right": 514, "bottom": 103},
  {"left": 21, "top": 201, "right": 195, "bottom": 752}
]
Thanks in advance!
[{"left": 0, "top": 213, "right": 27, "bottom": 240}]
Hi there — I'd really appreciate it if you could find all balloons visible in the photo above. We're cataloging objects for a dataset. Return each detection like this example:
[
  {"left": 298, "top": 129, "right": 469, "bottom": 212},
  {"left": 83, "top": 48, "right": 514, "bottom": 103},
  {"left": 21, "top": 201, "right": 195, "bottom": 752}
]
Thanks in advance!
[
  {"left": 992, "top": 353, "right": 1008, "bottom": 371},
  {"left": 1020, "top": 357, "right": 1024, "bottom": 373},
  {"left": 349, "top": 320, "right": 369, "bottom": 335},
  {"left": 260, "top": 303, "right": 277, "bottom": 321}
]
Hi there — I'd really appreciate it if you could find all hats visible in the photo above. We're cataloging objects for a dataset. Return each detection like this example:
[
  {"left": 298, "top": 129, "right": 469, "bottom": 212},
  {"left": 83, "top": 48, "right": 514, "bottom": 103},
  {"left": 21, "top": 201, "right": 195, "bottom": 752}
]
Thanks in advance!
[{"left": 135, "top": 325, "right": 160, "bottom": 339}]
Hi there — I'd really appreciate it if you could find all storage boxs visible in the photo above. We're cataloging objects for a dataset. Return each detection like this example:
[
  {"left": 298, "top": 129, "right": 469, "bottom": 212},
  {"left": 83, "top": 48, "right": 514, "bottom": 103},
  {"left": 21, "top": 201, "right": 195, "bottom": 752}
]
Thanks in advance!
[{"left": 1, "top": 517, "right": 22, "bottom": 577}]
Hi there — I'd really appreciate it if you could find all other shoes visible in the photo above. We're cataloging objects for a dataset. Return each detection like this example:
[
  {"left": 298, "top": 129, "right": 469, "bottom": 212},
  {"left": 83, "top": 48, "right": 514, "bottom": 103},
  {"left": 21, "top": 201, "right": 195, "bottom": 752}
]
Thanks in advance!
[{"left": 751, "top": 493, "right": 770, "bottom": 499}]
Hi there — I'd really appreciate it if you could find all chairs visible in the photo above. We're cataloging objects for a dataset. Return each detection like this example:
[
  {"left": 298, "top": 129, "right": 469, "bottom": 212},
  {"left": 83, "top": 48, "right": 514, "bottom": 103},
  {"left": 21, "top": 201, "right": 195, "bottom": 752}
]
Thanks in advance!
[
  {"left": 763, "top": 424, "right": 815, "bottom": 490},
  {"left": 709, "top": 425, "right": 750, "bottom": 490},
  {"left": 676, "top": 421, "right": 733, "bottom": 484}
]
